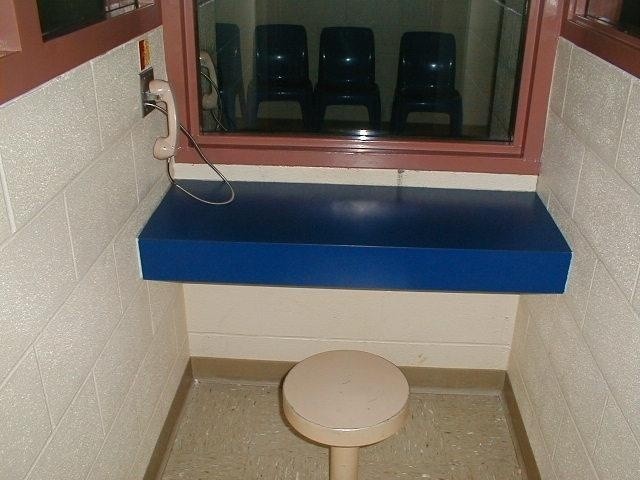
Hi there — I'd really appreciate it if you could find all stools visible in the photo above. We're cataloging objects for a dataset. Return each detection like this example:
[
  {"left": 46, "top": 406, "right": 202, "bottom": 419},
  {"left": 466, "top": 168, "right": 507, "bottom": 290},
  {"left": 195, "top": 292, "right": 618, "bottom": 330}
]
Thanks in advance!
[{"left": 282, "top": 350, "right": 410, "bottom": 480}]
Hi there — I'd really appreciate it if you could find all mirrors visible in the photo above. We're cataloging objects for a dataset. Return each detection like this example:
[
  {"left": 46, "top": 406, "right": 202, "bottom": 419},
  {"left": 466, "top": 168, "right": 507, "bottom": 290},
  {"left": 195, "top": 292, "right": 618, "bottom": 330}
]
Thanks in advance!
[{"left": 161, "top": 0, "right": 572, "bottom": 173}]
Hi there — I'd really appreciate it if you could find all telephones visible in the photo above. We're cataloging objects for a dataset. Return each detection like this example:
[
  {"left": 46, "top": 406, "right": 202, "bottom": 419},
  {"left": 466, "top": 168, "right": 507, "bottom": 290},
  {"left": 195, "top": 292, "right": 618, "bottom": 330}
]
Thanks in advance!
[
  {"left": 149, "top": 79, "right": 178, "bottom": 161},
  {"left": 200, "top": 51, "right": 219, "bottom": 110}
]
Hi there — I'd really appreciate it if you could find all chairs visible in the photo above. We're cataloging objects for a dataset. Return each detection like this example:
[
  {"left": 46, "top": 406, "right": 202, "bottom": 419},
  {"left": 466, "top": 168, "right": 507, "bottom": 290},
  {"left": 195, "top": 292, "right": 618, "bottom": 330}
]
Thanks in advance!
[
  {"left": 314, "top": 26, "right": 381, "bottom": 133},
  {"left": 251, "top": 23, "right": 313, "bottom": 131},
  {"left": 216, "top": 22, "right": 246, "bottom": 133},
  {"left": 390, "top": 30, "right": 461, "bottom": 136}
]
